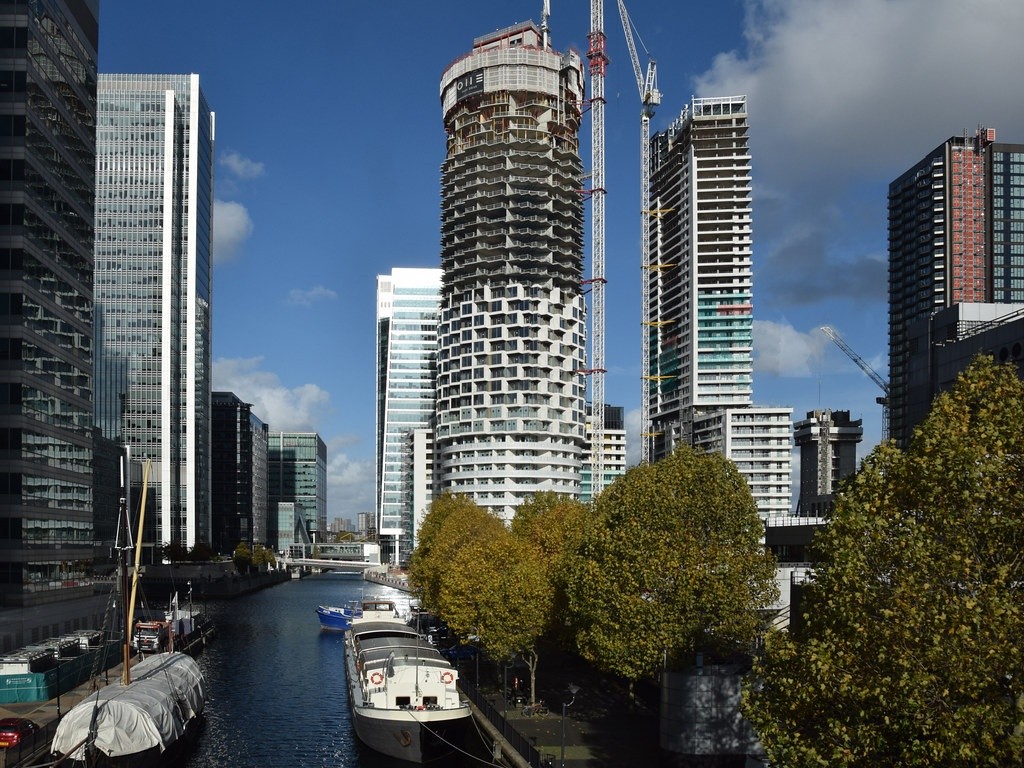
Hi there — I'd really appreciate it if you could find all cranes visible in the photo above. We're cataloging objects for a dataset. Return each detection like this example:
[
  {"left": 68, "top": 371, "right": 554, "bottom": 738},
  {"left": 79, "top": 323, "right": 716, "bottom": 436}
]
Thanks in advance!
[
  {"left": 612, "top": 0, "right": 663, "bottom": 461},
  {"left": 820, "top": 326, "right": 890, "bottom": 484}
]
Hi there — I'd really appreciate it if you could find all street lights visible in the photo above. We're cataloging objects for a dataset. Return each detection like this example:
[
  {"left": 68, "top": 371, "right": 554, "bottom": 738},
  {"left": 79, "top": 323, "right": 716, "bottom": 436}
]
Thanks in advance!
[{"left": 560, "top": 676, "right": 582, "bottom": 768}]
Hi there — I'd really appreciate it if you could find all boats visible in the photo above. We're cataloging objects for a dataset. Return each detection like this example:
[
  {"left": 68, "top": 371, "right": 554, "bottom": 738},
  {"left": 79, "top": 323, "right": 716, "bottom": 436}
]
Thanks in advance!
[
  {"left": 315, "top": 600, "right": 408, "bottom": 631},
  {"left": 345, "top": 618, "right": 473, "bottom": 763},
  {"left": 406, "top": 603, "right": 434, "bottom": 621}
]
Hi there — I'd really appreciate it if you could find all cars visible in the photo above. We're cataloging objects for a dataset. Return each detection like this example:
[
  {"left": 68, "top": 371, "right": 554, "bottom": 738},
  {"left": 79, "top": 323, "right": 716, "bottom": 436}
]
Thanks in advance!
[
  {"left": 0, "top": 718, "right": 40, "bottom": 750},
  {"left": 438, "top": 644, "right": 482, "bottom": 661}
]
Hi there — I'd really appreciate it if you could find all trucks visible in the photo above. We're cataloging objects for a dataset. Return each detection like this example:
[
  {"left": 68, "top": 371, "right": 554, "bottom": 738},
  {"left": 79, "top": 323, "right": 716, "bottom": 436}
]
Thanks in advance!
[{"left": 132, "top": 609, "right": 173, "bottom": 652}]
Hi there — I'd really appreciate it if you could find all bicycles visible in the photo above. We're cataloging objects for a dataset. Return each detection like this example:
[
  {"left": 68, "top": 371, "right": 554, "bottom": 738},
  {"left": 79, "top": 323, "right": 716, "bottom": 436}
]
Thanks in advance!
[{"left": 522, "top": 700, "right": 550, "bottom": 718}]
